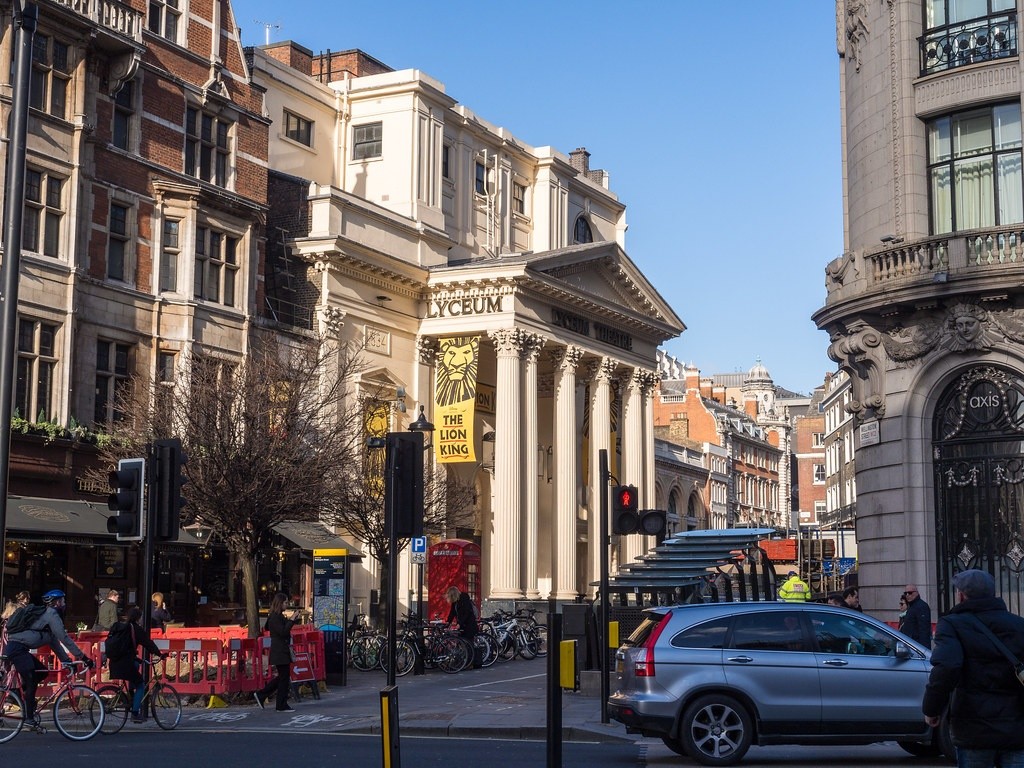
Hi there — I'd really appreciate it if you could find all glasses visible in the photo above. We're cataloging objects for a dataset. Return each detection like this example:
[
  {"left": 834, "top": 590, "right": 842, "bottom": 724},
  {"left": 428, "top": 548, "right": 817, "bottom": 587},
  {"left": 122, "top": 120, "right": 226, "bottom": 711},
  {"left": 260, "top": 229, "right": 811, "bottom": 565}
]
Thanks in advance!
[
  {"left": 904, "top": 591, "right": 917, "bottom": 595},
  {"left": 899, "top": 602, "right": 907, "bottom": 606}
]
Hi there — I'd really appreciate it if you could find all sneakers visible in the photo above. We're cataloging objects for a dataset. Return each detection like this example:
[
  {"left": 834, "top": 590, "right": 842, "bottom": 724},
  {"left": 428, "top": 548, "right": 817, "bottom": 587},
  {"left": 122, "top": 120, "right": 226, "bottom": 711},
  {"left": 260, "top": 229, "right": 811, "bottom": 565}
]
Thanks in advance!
[
  {"left": 276, "top": 704, "right": 296, "bottom": 712},
  {"left": 254, "top": 692, "right": 265, "bottom": 709}
]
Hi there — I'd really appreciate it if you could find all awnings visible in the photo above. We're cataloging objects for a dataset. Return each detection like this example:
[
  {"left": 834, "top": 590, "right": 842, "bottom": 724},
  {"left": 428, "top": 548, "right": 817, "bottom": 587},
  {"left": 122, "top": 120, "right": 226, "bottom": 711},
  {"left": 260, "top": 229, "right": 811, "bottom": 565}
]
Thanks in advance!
[
  {"left": 268, "top": 519, "right": 366, "bottom": 558},
  {"left": 5, "top": 496, "right": 228, "bottom": 547}
]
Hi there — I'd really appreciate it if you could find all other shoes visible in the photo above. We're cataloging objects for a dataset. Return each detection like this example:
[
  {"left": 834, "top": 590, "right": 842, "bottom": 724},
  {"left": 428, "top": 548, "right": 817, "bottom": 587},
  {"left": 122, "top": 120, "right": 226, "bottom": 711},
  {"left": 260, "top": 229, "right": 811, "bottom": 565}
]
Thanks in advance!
[
  {"left": 8, "top": 690, "right": 21, "bottom": 702},
  {"left": 24, "top": 718, "right": 38, "bottom": 728},
  {"left": 465, "top": 665, "right": 482, "bottom": 670},
  {"left": 131, "top": 713, "right": 147, "bottom": 722}
]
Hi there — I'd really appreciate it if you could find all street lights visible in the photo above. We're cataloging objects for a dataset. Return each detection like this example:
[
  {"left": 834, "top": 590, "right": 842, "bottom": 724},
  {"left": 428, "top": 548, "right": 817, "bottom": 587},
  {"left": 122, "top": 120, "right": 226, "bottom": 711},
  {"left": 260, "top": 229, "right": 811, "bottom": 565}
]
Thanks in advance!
[{"left": 409, "top": 405, "right": 436, "bottom": 676}]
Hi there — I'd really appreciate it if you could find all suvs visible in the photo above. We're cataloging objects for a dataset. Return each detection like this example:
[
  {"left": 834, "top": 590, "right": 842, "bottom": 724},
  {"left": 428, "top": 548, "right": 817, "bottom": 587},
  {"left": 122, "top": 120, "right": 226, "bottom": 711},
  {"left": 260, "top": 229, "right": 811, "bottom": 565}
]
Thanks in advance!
[{"left": 607, "top": 599, "right": 935, "bottom": 768}]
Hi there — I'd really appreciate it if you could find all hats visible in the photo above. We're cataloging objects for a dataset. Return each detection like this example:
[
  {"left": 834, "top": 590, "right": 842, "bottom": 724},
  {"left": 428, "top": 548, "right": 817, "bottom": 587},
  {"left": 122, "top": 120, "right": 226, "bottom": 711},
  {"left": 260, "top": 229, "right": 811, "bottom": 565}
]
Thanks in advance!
[{"left": 950, "top": 569, "right": 996, "bottom": 599}]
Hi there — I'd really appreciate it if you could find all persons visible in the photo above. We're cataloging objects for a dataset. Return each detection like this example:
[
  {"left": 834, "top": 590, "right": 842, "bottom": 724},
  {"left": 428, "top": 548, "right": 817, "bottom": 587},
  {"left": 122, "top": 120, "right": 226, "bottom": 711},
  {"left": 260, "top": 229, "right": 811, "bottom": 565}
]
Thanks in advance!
[
  {"left": 0, "top": 591, "right": 30, "bottom": 711},
  {"left": 6, "top": 591, "right": 93, "bottom": 727},
  {"left": 899, "top": 585, "right": 931, "bottom": 650},
  {"left": 109, "top": 609, "right": 168, "bottom": 722},
  {"left": 942, "top": 303, "right": 1005, "bottom": 351},
  {"left": 140, "top": 593, "right": 172, "bottom": 632},
  {"left": 445, "top": 587, "right": 477, "bottom": 670},
  {"left": 922, "top": 570, "right": 1024, "bottom": 768},
  {"left": 254, "top": 594, "right": 301, "bottom": 712},
  {"left": 844, "top": 586, "right": 863, "bottom": 612},
  {"left": 779, "top": 571, "right": 811, "bottom": 602},
  {"left": 680, "top": 585, "right": 704, "bottom": 603},
  {"left": 98, "top": 590, "right": 119, "bottom": 630},
  {"left": 827, "top": 595, "right": 848, "bottom": 607}
]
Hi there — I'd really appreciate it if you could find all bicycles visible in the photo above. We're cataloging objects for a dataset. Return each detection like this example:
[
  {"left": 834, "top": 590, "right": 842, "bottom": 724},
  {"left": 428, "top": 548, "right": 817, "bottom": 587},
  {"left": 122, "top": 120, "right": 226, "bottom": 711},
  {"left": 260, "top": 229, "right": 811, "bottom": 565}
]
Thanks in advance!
[
  {"left": 0, "top": 655, "right": 106, "bottom": 743},
  {"left": 88, "top": 653, "right": 181, "bottom": 736},
  {"left": 347, "top": 605, "right": 547, "bottom": 677}
]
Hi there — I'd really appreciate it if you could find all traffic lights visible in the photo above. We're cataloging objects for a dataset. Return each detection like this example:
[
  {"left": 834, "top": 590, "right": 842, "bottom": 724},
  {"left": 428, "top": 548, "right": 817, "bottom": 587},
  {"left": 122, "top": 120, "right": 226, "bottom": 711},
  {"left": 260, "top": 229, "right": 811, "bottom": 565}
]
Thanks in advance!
[
  {"left": 641, "top": 510, "right": 666, "bottom": 534},
  {"left": 614, "top": 486, "right": 638, "bottom": 534},
  {"left": 176, "top": 453, "right": 189, "bottom": 509},
  {"left": 107, "top": 469, "right": 135, "bottom": 533}
]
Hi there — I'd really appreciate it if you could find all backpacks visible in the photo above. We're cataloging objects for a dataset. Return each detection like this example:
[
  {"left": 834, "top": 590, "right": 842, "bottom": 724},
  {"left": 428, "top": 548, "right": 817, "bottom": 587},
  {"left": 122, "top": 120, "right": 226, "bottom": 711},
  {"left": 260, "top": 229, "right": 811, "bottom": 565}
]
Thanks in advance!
[
  {"left": 104, "top": 622, "right": 133, "bottom": 657},
  {"left": 6, "top": 604, "right": 51, "bottom": 634},
  {"left": 470, "top": 598, "right": 481, "bottom": 619}
]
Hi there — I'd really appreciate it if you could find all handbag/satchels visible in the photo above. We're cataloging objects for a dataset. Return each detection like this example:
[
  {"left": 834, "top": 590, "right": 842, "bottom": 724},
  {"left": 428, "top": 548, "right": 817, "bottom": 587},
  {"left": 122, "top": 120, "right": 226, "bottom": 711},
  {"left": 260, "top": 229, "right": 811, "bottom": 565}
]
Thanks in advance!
[
  {"left": 91, "top": 623, "right": 111, "bottom": 632},
  {"left": 1014, "top": 662, "right": 1024, "bottom": 687}
]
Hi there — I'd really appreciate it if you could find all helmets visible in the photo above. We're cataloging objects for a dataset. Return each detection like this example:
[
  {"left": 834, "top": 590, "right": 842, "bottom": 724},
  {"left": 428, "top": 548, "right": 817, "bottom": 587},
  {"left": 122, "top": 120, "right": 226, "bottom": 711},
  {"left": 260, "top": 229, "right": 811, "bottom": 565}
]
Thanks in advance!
[
  {"left": 788, "top": 571, "right": 795, "bottom": 579},
  {"left": 42, "top": 590, "right": 65, "bottom": 603}
]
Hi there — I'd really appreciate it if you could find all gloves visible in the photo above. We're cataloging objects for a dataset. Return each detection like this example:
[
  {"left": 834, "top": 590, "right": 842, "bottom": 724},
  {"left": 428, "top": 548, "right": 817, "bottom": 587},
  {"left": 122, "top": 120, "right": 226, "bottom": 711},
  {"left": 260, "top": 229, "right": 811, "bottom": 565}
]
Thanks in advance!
[
  {"left": 65, "top": 660, "right": 77, "bottom": 673},
  {"left": 82, "top": 654, "right": 93, "bottom": 669}
]
